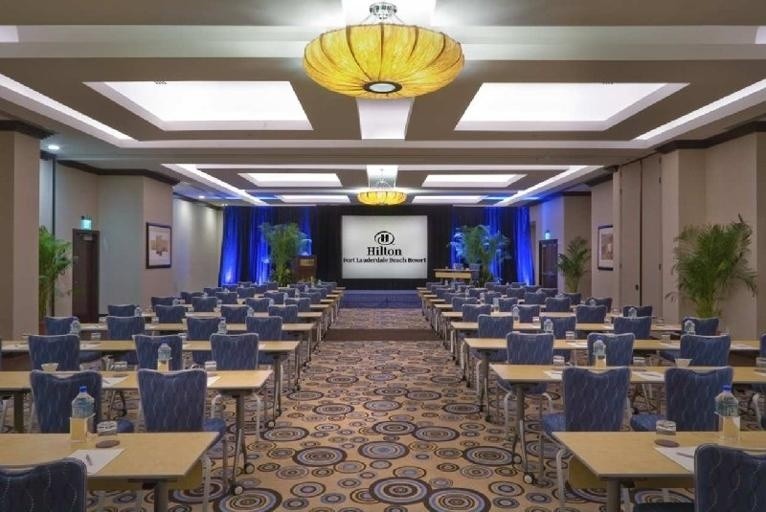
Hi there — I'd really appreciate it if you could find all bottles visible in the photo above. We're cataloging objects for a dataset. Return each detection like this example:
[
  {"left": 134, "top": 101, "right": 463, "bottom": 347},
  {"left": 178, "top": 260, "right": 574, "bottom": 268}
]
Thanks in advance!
[
  {"left": 218, "top": 318, "right": 227, "bottom": 334},
  {"left": 134, "top": 306, "right": 141, "bottom": 318},
  {"left": 70, "top": 316, "right": 80, "bottom": 337},
  {"left": 714, "top": 384, "right": 742, "bottom": 449},
  {"left": 493, "top": 299, "right": 499, "bottom": 316},
  {"left": 268, "top": 297, "right": 274, "bottom": 308},
  {"left": 172, "top": 298, "right": 179, "bottom": 305},
  {"left": 69, "top": 386, "right": 93, "bottom": 456},
  {"left": 157, "top": 340, "right": 171, "bottom": 373},
  {"left": 283, "top": 292, "right": 288, "bottom": 304},
  {"left": 544, "top": 317, "right": 554, "bottom": 335},
  {"left": 304, "top": 285, "right": 309, "bottom": 294},
  {"left": 480, "top": 293, "right": 485, "bottom": 305},
  {"left": 592, "top": 335, "right": 607, "bottom": 372},
  {"left": 628, "top": 305, "right": 636, "bottom": 320},
  {"left": 684, "top": 317, "right": 695, "bottom": 337},
  {"left": 247, "top": 306, "right": 254, "bottom": 318},
  {"left": 107, "top": 356, "right": 116, "bottom": 379},
  {"left": 295, "top": 288, "right": 299, "bottom": 298},
  {"left": 512, "top": 305, "right": 519, "bottom": 329}
]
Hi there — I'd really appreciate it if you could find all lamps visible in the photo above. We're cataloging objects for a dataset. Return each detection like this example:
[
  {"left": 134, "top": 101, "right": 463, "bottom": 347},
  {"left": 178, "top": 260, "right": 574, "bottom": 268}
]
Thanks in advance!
[
  {"left": 356, "top": 181, "right": 409, "bottom": 208},
  {"left": 301, "top": 5, "right": 465, "bottom": 101}
]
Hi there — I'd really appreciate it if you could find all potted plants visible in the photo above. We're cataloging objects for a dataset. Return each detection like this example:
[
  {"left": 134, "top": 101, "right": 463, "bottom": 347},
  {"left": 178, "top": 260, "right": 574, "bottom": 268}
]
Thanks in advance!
[{"left": 39, "top": 226, "right": 78, "bottom": 336}]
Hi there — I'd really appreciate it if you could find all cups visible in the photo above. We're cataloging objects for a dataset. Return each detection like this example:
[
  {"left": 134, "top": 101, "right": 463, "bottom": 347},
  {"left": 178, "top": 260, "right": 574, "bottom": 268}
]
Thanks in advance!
[
  {"left": 41, "top": 363, "right": 58, "bottom": 375},
  {"left": 204, "top": 361, "right": 217, "bottom": 378},
  {"left": 675, "top": 358, "right": 691, "bottom": 369},
  {"left": 723, "top": 416, "right": 740, "bottom": 447},
  {"left": 633, "top": 357, "right": 646, "bottom": 372},
  {"left": 756, "top": 358, "right": 766, "bottom": 375},
  {"left": 70, "top": 416, "right": 88, "bottom": 443},
  {"left": 552, "top": 356, "right": 564, "bottom": 376},
  {"left": 114, "top": 361, "right": 127, "bottom": 377},
  {"left": 565, "top": 331, "right": 575, "bottom": 345},
  {"left": 91, "top": 332, "right": 101, "bottom": 345},
  {"left": 157, "top": 359, "right": 170, "bottom": 374},
  {"left": 150, "top": 316, "right": 160, "bottom": 327},
  {"left": 656, "top": 419, "right": 677, "bottom": 449},
  {"left": 97, "top": 421, "right": 117, "bottom": 447}
]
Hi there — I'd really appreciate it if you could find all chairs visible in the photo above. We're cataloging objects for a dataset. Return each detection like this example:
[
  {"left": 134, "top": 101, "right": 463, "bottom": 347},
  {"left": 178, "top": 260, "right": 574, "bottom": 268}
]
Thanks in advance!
[
  {"left": 247, "top": 299, "right": 271, "bottom": 313},
  {"left": 29, "top": 336, "right": 80, "bottom": 373},
  {"left": 625, "top": 306, "right": 654, "bottom": 316},
  {"left": 152, "top": 298, "right": 170, "bottom": 310},
  {"left": 431, "top": 285, "right": 446, "bottom": 292},
  {"left": 279, "top": 288, "right": 297, "bottom": 296},
  {"left": 457, "top": 286, "right": 471, "bottom": 292},
  {"left": 697, "top": 447, "right": 766, "bottom": 512},
  {"left": 106, "top": 317, "right": 144, "bottom": 365},
  {"left": 485, "top": 282, "right": 503, "bottom": 289},
  {"left": 216, "top": 292, "right": 235, "bottom": 304},
  {"left": 565, "top": 294, "right": 581, "bottom": 302},
  {"left": 192, "top": 298, "right": 216, "bottom": 312},
  {"left": 300, "top": 293, "right": 320, "bottom": 304},
  {"left": 238, "top": 287, "right": 255, "bottom": 298},
  {"left": 587, "top": 297, "right": 612, "bottom": 312},
  {"left": 539, "top": 317, "right": 576, "bottom": 360},
  {"left": 525, "top": 286, "right": 537, "bottom": 293},
  {"left": 512, "top": 282, "right": 527, "bottom": 289},
  {"left": 238, "top": 282, "right": 251, "bottom": 288},
  {"left": 221, "top": 284, "right": 237, "bottom": 293},
  {"left": 682, "top": 334, "right": 730, "bottom": 369},
  {"left": 454, "top": 298, "right": 476, "bottom": 308},
  {"left": 587, "top": 333, "right": 633, "bottom": 368},
  {"left": 182, "top": 292, "right": 204, "bottom": 304},
  {"left": 283, "top": 299, "right": 311, "bottom": 311},
  {"left": 215, "top": 293, "right": 236, "bottom": 303},
  {"left": 322, "top": 282, "right": 336, "bottom": 288},
  {"left": 28, "top": 371, "right": 101, "bottom": 430},
  {"left": 137, "top": 370, "right": 228, "bottom": 498},
  {"left": 246, "top": 315, "right": 292, "bottom": 394},
  {"left": 317, "top": 285, "right": 332, "bottom": 291},
  {"left": 221, "top": 306, "right": 247, "bottom": 324},
  {"left": 269, "top": 305, "right": 302, "bottom": 340},
  {"left": 204, "top": 289, "right": 221, "bottom": 298},
  {"left": 493, "top": 285, "right": 512, "bottom": 294},
  {"left": 471, "top": 317, "right": 513, "bottom": 398},
  {"left": 631, "top": 369, "right": 735, "bottom": 432},
  {"left": 576, "top": 307, "right": 607, "bottom": 340},
  {"left": 108, "top": 305, "right": 135, "bottom": 317},
  {"left": 297, "top": 281, "right": 311, "bottom": 289},
  {"left": 454, "top": 281, "right": 464, "bottom": 289},
  {"left": 541, "top": 367, "right": 630, "bottom": 509},
  {"left": 507, "top": 288, "right": 526, "bottom": 296},
  {"left": 539, "top": 288, "right": 558, "bottom": 298},
  {"left": 251, "top": 285, "right": 267, "bottom": 294},
  {"left": 188, "top": 318, "right": 218, "bottom": 365},
  {"left": 670, "top": 319, "right": 718, "bottom": 339},
  {"left": 181, "top": 292, "right": 201, "bottom": 305},
  {"left": 426, "top": 281, "right": 441, "bottom": 289},
  {"left": 133, "top": 335, "right": 182, "bottom": 370},
  {"left": 460, "top": 305, "right": 488, "bottom": 338},
  {"left": 469, "top": 288, "right": 487, "bottom": 299},
  {"left": 760, "top": 334, "right": 766, "bottom": 356},
  {"left": 309, "top": 288, "right": 325, "bottom": 297},
  {"left": 484, "top": 292, "right": 502, "bottom": 304},
  {"left": 0, "top": 458, "right": 89, "bottom": 512},
  {"left": 526, "top": 293, "right": 545, "bottom": 303},
  {"left": 264, "top": 282, "right": 279, "bottom": 290},
  {"left": 448, "top": 293, "right": 464, "bottom": 303},
  {"left": 498, "top": 298, "right": 517, "bottom": 311},
  {"left": 43, "top": 316, "right": 104, "bottom": 364},
  {"left": 514, "top": 305, "right": 539, "bottom": 333},
  {"left": 497, "top": 332, "right": 553, "bottom": 436},
  {"left": 438, "top": 289, "right": 452, "bottom": 296},
  {"left": 156, "top": 306, "right": 184, "bottom": 334},
  {"left": 545, "top": 299, "right": 569, "bottom": 313},
  {"left": 615, "top": 317, "right": 651, "bottom": 358},
  {"left": 211, "top": 334, "right": 267, "bottom": 440},
  {"left": 301, "top": 293, "right": 320, "bottom": 303}
]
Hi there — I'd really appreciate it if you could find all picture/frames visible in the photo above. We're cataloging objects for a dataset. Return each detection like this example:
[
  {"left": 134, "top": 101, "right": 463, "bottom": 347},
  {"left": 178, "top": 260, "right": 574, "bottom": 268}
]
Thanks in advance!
[
  {"left": 597, "top": 226, "right": 615, "bottom": 270},
  {"left": 146, "top": 223, "right": 172, "bottom": 268}
]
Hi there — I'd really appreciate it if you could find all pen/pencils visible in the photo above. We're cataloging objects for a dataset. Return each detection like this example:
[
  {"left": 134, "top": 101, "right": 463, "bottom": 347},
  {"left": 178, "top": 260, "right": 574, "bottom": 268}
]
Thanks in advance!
[{"left": 86, "top": 454, "right": 94, "bottom": 467}]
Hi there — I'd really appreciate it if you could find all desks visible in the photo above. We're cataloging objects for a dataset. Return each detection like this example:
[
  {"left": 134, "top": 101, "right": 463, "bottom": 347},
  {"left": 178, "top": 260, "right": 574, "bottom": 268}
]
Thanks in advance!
[
  {"left": 417, "top": 286, "right": 463, "bottom": 345},
  {"left": 80, "top": 322, "right": 315, "bottom": 363},
  {"left": 1, "top": 339, "right": 299, "bottom": 428},
  {"left": 175, "top": 303, "right": 330, "bottom": 335},
  {"left": 433, "top": 270, "right": 481, "bottom": 284},
  {"left": 0, "top": 430, "right": 221, "bottom": 512},
  {"left": 489, "top": 363, "right": 766, "bottom": 485},
  {"left": 293, "top": 256, "right": 316, "bottom": 282},
  {"left": 140, "top": 310, "right": 322, "bottom": 350},
  {"left": 236, "top": 298, "right": 334, "bottom": 323},
  {"left": 550, "top": 430, "right": 766, "bottom": 512},
  {"left": 462, "top": 337, "right": 766, "bottom": 423},
  {"left": 1, "top": 370, "right": 274, "bottom": 495},
  {"left": 447, "top": 321, "right": 682, "bottom": 361}
]
